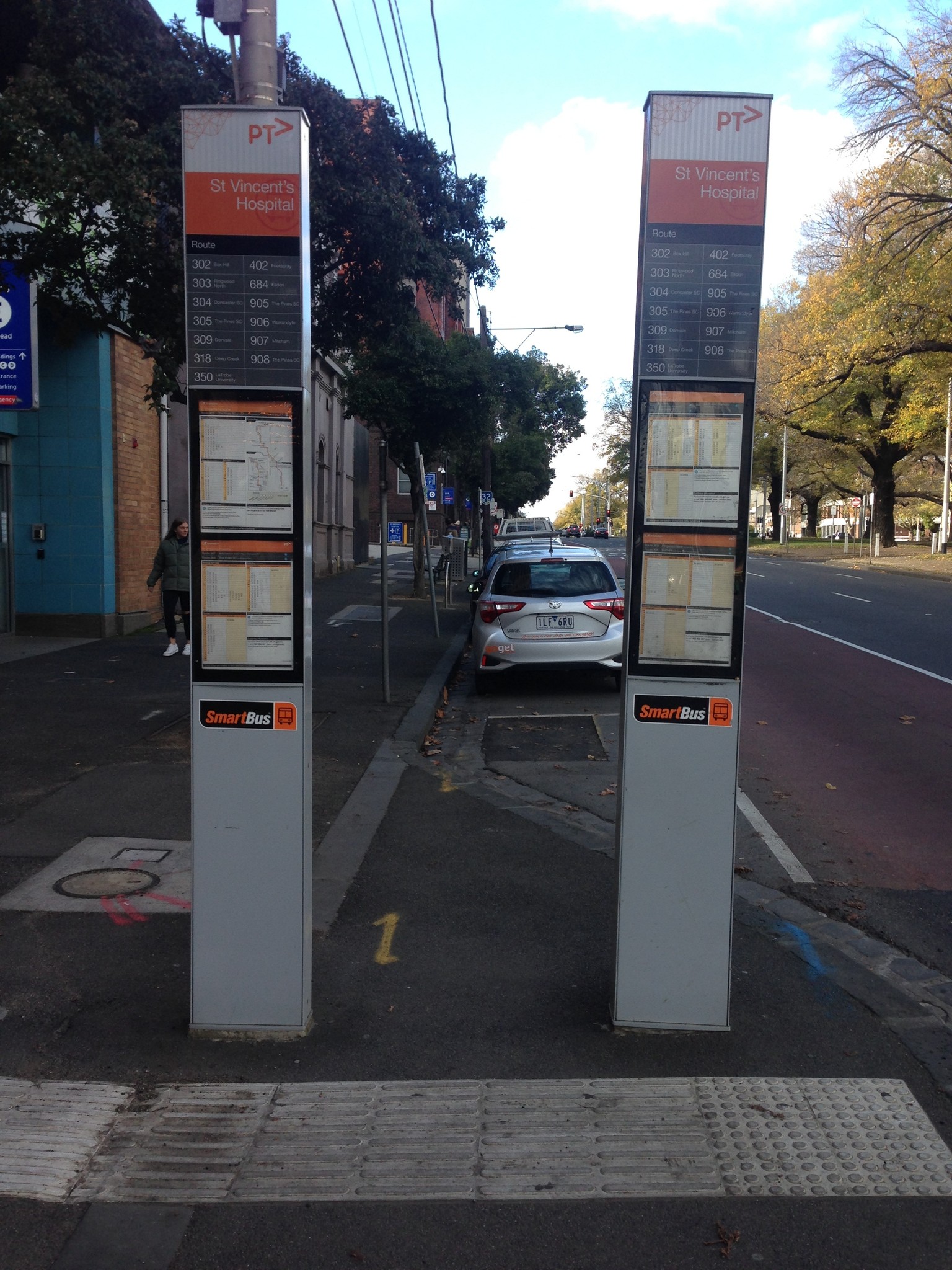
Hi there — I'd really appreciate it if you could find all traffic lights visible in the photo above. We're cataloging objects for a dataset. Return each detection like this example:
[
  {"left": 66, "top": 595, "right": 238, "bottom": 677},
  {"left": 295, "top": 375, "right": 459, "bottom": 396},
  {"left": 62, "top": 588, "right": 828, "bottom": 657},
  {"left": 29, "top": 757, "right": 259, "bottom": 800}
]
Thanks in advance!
[
  {"left": 606, "top": 510, "right": 610, "bottom": 517},
  {"left": 569, "top": 490, "right": 574, "bottom": 498},
  {"left": 596, "top": 518, "right": 600, "bottom": 525},
  {"left": 580, "top": 524, "right": 583, "bottom": 528}
]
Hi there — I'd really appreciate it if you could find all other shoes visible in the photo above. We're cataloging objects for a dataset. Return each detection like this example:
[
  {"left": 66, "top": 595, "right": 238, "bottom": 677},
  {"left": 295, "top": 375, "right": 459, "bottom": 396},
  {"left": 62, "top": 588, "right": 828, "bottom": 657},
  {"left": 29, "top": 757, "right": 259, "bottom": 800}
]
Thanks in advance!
[
  {"left": 163, "top": 643, "right": 180, "bottom": 657},
  {"left": 181, "top": 644, "right": 191, "bottom": 655}
]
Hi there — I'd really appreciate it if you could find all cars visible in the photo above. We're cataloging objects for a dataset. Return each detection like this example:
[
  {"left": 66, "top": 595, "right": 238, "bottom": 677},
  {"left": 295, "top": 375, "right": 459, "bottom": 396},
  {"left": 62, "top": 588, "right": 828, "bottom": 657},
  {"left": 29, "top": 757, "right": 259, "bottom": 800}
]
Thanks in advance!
[
  {"left": 465, "top": 516, "right": 625, "bottom": 697},
  {"left": 824, "top": 531, "right": 853, "bottom": 540},
  {"left": 593, "top": 528, "right": 609, "bottom": 539},
  {"left": 566, "top": 525, "right": 581, "bottom": 538}
]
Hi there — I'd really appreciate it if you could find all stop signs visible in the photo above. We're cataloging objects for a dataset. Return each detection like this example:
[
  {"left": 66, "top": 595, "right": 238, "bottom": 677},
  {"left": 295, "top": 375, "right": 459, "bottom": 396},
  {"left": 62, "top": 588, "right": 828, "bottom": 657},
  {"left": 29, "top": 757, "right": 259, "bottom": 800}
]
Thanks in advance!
[{"left": 852, "top": 496, "right": 861, "bottom": 507}]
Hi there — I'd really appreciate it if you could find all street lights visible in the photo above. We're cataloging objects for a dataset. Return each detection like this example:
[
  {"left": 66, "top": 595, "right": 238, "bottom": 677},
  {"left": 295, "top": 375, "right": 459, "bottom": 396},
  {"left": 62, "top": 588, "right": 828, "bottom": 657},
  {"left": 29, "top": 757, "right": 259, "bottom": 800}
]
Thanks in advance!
[
  {"left": 477, "top": 304, "right": 585, "bottom": 565},
  {"left": 571, "top": 476, "right": 601, "bottom": 518}
]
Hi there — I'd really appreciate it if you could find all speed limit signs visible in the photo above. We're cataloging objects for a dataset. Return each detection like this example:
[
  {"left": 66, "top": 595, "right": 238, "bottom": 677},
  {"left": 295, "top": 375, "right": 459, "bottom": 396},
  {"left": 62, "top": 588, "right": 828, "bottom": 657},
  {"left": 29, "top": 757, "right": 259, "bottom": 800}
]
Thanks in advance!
[{"left": 778, "top": 503, "right": 789, "bottom": 516}]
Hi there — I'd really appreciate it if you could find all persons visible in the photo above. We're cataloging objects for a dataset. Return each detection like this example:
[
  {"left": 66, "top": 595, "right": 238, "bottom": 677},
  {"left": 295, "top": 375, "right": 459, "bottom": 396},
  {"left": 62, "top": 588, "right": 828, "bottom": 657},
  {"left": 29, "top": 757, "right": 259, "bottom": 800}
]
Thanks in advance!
[
  {"left": 146, "top": 517, "right": 191, "bottom": 656},
  {"left": 444, "top": 518, "right": 460, "bottom": 537}
]
